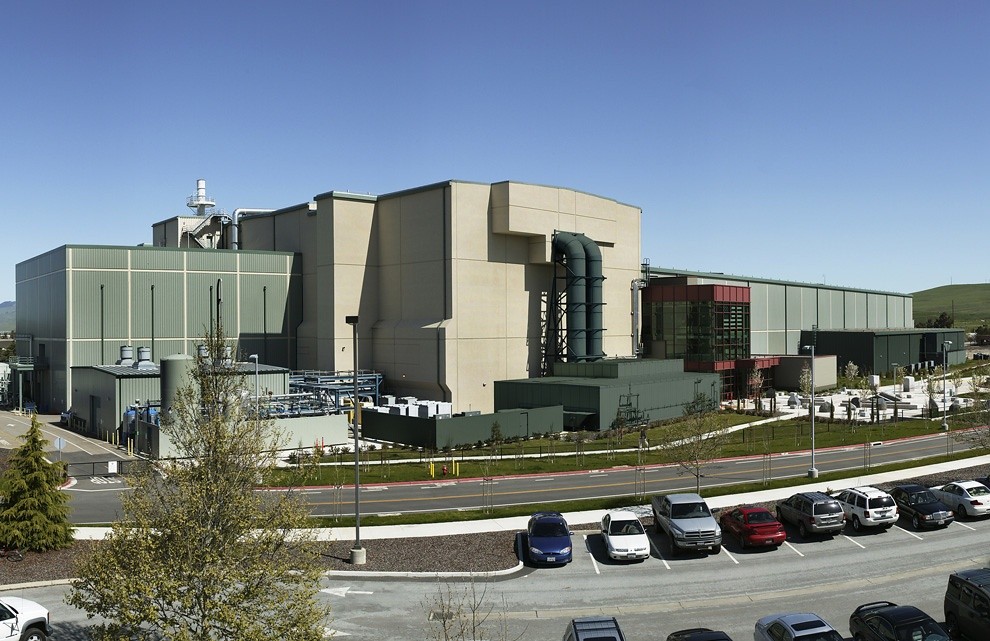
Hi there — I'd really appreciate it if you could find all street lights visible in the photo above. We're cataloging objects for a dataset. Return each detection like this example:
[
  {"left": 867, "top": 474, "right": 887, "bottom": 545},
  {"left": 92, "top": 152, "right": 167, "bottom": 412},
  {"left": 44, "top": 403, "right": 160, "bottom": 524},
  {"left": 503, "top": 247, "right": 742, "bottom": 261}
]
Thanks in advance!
[
  {"left": 941, "top": 340, "right": 952, "bottom": 431},
  {"left": 892, "top": 362, "right": 900, "bottom": 421},
  {"left": 802, "top": 344, "right": 819, "bottom": 480},
  {"left": 248, "top": 353, "right": 258, "bottom": 475},
  {"left": 344, "top": 314, "right": 367, "bottom": 566}
]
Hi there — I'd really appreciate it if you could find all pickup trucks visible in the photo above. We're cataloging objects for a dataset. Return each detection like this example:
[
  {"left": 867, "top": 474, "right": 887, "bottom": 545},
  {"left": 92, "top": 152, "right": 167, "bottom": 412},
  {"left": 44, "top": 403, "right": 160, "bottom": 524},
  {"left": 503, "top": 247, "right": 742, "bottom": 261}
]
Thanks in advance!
[{"left": 650, "top": 492, "right": 723, "bottom": 556}]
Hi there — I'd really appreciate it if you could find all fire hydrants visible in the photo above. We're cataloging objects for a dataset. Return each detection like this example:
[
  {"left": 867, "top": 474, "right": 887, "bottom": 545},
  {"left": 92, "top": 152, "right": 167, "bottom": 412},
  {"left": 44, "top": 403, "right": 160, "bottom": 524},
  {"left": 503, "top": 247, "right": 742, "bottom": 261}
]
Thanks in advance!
[{"left": 441, "top": 464, "right": 448, "bottom": 477}]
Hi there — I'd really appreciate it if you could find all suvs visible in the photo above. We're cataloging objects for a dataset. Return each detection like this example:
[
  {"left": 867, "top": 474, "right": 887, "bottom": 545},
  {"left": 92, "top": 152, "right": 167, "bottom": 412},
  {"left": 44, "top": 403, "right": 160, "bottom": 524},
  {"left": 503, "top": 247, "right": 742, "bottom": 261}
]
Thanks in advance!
[
  {"left": 776, "top": 491, "right": 846, "bottom": 539},
  {"left": 828, "top": 485, "right": 900, "bottom": 531}
]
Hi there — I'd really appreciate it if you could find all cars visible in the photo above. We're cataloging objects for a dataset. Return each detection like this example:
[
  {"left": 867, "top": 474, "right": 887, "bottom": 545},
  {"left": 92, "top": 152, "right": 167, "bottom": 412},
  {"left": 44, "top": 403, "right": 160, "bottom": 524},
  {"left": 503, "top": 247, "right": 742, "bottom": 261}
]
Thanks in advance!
[
  {"left": 720, "top": 505, "right": 787, "bottom": 549},
  {"left": 600, "top": 511, "right": 651, "bottom": 561},
  {"left": 753, "top": 611, "right": 846, "bottom": 641},
  {"left": 928, "top": 480, "right": 990, "bottom": 519},
  {"left": 884, "top": 483, "right": 956, "bottom": 531},
  {"left": 527, "top": 510, "right": 575, "bottom": 567},
  {"left": 0, "top": 595, "right": 54, "bottom": 641},
  {"left": 848, "top": 601, "right": 953, "bottom": 641},
  {"left": 975, "top": 473, "right": 990, "bottom": 489},
  {"left": 666, "top": 626, "right": 732, "bottom": 641}
]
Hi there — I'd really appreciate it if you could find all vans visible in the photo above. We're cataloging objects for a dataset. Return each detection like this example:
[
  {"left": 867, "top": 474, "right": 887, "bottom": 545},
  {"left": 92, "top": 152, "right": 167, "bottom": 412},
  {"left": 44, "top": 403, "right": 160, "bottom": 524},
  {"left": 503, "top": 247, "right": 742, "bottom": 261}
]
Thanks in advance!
[
  {"left": 562, "top": 616, "right": 627, "bottom": 641},
  {"left": 942, "top": 567, "right": 990, "bottom": 641}
]
